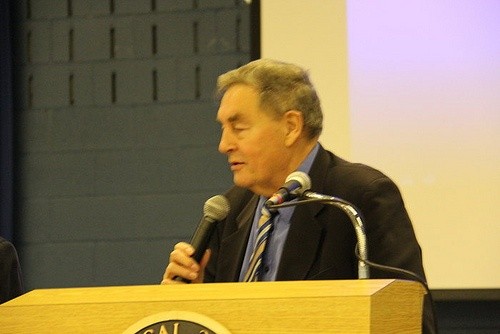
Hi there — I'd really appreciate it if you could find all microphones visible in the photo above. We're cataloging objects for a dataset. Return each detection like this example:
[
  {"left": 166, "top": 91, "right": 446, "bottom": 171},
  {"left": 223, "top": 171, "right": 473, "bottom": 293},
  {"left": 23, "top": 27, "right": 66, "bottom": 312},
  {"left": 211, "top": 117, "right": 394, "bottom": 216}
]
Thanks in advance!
[
  {"left": 264, "top": 171, "right": 312, "bottom": 209},
  {"left": 171, "top": 195, "right": 231, "bottom": 283}
]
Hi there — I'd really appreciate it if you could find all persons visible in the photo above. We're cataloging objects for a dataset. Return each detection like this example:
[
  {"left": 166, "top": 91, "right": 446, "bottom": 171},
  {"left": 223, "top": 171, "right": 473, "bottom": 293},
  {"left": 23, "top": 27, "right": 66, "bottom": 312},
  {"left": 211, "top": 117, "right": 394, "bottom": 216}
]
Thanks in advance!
[{"left": 162, "top": 59, "right": 436, "bottom": 333}]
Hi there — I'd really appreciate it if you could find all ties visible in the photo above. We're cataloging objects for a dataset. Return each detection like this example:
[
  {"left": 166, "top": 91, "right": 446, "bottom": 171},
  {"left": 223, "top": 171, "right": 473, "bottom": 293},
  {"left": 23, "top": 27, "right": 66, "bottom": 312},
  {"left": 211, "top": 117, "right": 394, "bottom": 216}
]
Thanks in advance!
[{"left": 244, "top": 205, "right": 279, "bottom": 282}]
